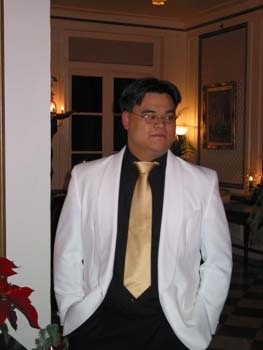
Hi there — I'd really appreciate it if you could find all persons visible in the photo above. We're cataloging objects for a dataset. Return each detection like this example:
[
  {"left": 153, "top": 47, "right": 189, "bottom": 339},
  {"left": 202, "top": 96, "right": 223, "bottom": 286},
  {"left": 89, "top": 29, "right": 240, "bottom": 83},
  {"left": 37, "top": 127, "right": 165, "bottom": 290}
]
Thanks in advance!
[{"left": 52, "top": 76, "right": 234, "bottom": 350}]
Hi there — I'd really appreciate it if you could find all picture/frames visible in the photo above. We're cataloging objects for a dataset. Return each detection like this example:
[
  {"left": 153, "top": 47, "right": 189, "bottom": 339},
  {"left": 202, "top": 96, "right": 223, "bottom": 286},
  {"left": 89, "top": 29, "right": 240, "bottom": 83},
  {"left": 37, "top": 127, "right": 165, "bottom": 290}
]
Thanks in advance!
[{"left": 199, "top": 80, "right": 237, "bottom": 152}]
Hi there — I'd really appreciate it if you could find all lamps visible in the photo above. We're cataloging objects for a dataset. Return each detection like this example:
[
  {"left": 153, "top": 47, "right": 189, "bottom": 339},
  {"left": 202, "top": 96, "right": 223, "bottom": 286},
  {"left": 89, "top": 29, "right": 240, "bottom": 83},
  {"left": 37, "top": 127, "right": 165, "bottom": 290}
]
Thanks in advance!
[
  {"left": 242, "top": 167, "right": 263, "bottom": 192},
  {"left": 170, "top": 108, "right": 196, "bottom": 161},
  {"left": 50, "top": 76, "right": 74, "bottom": 185}
]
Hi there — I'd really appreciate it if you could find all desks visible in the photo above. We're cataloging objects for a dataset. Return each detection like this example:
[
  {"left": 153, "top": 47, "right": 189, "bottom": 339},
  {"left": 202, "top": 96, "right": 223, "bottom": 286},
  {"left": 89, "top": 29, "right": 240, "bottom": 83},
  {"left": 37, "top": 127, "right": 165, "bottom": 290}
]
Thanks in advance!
[{"left": 225, "top": 201, "right": 263, "bottom": 271}]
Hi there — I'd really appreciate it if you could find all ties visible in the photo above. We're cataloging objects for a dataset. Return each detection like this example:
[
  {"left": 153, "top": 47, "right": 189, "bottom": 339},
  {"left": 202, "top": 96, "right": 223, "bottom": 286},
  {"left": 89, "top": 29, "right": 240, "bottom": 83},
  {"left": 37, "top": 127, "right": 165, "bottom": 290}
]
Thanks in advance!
[{"left": 124, "top": 161, "right": 155, "bottom": 299}]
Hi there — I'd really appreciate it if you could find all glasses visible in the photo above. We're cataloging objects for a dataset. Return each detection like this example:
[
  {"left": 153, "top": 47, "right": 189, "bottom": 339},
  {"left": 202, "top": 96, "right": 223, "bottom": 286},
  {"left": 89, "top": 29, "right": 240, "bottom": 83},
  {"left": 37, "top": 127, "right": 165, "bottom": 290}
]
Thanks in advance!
[{"left": 128, "top": 111, "right": 176, "bottom": 124}]
